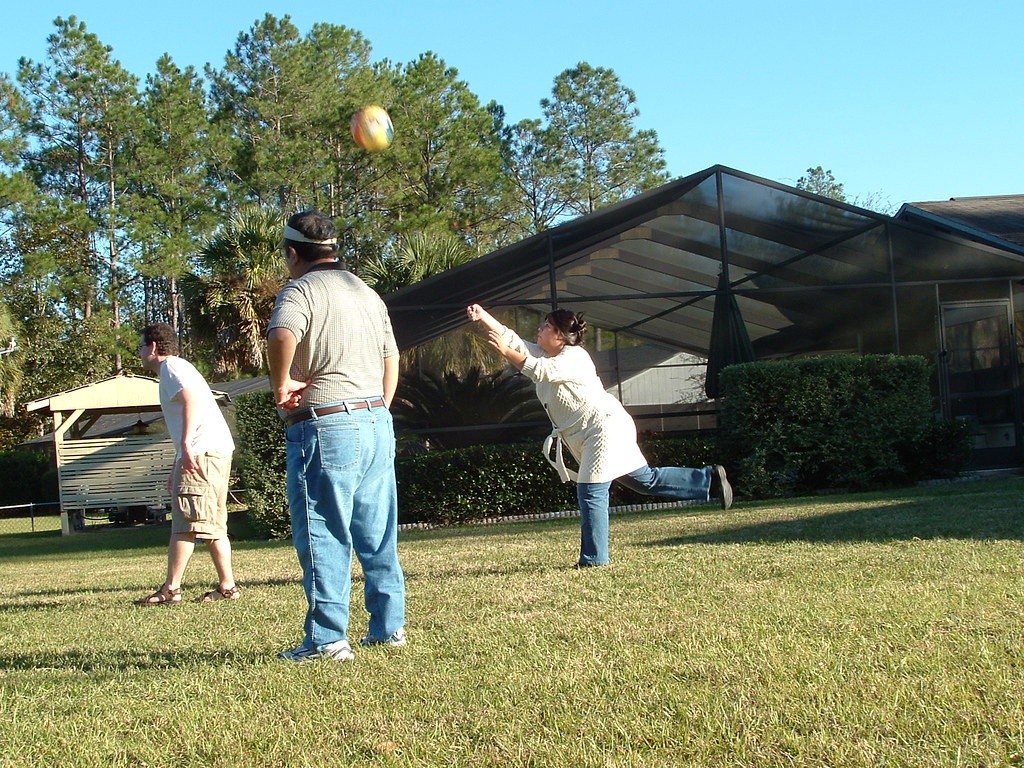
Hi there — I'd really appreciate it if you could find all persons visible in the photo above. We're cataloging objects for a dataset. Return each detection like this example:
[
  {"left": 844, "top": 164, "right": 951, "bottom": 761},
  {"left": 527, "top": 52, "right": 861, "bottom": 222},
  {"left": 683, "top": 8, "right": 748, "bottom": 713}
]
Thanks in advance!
[
  {"left": 137, "top": 322, "right": 240, "bottom": 606},
  {"left": 265, "top": 210, "right": 407, "bottom": 661},
  {"left": 466, "top": 303, "right": 733, "bottom": 569}
]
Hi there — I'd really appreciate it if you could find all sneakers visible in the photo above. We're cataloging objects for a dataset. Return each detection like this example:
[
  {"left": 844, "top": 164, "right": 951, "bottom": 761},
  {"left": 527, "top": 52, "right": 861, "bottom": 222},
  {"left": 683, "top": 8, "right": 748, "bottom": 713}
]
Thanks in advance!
[
  {"left": 279, "top": 639, "right": 354, "bottom": 660},
  {"left": 360, "top": 626, "right": 406, "bottom": 646}
]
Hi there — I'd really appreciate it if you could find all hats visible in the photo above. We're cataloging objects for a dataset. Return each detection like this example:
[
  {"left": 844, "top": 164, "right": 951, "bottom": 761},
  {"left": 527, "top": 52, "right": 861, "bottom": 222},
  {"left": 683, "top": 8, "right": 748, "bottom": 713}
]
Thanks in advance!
[{"left": 274, "top": 227, "right": 337, "bottom": 251}]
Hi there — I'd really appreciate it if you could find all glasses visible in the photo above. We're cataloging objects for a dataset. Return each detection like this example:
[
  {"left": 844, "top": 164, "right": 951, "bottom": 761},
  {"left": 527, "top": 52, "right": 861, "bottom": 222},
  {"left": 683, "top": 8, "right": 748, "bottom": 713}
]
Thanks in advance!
[{"left": 137, "top": 342, "right": 153, "bottom": 352}]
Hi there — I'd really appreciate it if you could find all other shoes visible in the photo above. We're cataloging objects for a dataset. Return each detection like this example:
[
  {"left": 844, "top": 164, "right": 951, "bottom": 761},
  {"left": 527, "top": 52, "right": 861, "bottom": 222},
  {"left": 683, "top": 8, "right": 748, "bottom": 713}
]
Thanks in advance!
[
  {"left": 574, "top": 562, "right": 591, "bottom": 569},
  {"left": 710, "top": 464, "right": 732, "bottom": 510}
]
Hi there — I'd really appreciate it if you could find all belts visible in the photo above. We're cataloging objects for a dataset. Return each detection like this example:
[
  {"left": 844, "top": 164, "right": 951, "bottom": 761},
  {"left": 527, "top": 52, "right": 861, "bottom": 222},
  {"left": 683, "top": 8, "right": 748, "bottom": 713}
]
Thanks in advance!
[{"left": 286, "top": 399, "right": 385, "bottom": 426}]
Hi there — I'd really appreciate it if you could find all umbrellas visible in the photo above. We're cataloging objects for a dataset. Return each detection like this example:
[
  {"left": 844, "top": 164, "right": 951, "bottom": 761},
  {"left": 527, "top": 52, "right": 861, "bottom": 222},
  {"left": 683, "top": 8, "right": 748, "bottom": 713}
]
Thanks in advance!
[{"left": 705, "top": 275, "right": 758, "bottom": 399}]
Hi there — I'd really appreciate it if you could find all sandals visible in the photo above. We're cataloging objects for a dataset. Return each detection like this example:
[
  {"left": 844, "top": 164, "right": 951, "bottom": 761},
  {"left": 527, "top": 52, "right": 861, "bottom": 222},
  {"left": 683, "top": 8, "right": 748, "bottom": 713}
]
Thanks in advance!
[
  {"left": 191, "top": 586, "right": 238, "bottom": 603},
  {"left": 133, "top": 584, "right": 183, "bottom": 605}
]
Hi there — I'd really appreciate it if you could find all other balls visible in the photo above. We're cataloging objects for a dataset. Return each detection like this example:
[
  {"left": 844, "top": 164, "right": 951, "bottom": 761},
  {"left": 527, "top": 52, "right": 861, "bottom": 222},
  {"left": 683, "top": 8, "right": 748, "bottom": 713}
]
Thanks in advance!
[{"left": 350, "top": 106, "right": 395, "bottom": 154}]
